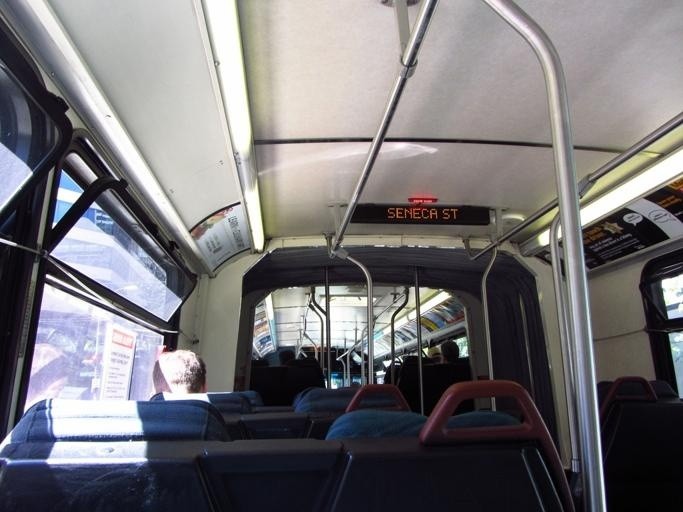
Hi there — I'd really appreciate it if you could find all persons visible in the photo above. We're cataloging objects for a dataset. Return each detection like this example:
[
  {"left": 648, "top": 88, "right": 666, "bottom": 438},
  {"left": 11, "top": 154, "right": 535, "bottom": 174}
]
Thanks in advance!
[
  {"left": 439, "top": 339, "right": 459, "bottom": 364},
  {"left": 150, "top": 348, "right": 206, "bottom": 396}
]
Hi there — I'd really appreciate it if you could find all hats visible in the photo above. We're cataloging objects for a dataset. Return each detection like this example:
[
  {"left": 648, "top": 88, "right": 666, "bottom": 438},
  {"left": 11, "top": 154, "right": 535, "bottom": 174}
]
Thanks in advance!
[
  {"left": 442, "top": 341, "right": 459, "bottom": 358},
  {"left": 428, "top": 347, "right": 439, "bottom": 355}
]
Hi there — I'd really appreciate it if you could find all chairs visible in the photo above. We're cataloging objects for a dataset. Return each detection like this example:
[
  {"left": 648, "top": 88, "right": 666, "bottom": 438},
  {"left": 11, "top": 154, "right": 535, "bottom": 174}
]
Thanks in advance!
[
  {"left": 596, "top": 377, "right": 682, "bottom": 511},
  {"left": 250, "top": 357, "right": 474, "bottom": 418}
]
